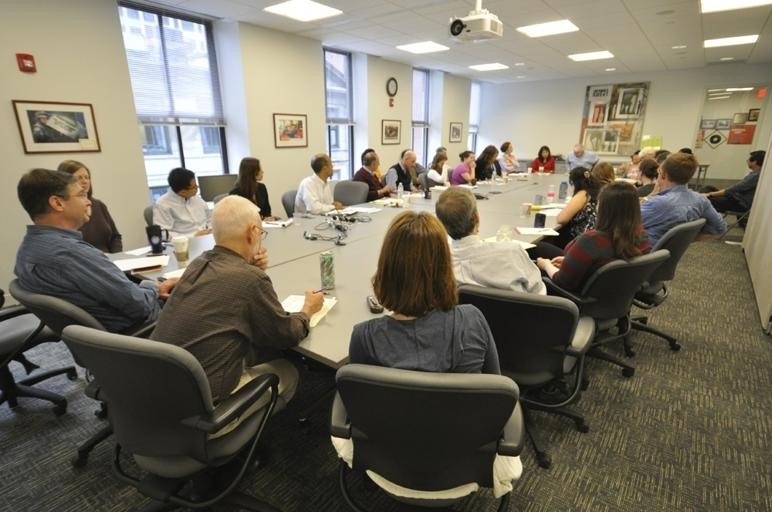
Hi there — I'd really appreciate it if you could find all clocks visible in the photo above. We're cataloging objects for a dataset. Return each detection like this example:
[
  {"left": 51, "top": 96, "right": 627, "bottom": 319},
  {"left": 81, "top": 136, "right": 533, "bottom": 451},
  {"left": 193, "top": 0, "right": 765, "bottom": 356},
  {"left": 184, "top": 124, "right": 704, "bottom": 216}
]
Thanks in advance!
[{"left": 387, "top": 77, "right": 397, "bottom": 97}]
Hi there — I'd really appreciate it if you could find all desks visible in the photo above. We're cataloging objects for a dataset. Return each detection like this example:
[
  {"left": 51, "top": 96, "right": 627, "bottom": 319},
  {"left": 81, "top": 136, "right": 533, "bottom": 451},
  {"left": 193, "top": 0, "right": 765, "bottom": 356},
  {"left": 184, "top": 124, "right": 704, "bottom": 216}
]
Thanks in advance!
[{"left": 100, "top": 172, "right": 639, "bottom": 369}]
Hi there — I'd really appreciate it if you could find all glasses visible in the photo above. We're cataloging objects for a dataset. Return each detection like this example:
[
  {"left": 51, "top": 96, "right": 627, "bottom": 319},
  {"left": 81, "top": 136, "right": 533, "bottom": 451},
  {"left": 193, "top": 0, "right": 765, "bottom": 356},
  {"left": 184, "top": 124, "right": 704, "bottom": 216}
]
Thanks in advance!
[
  {"left": 187, "top": 184, "right": 197, "bottom": 189},
  {"left": 58, "top": 192, "right": 89, "bottom": 201},
  {"left": 254, "top": 225, "right": 268, "bottom": 241}
]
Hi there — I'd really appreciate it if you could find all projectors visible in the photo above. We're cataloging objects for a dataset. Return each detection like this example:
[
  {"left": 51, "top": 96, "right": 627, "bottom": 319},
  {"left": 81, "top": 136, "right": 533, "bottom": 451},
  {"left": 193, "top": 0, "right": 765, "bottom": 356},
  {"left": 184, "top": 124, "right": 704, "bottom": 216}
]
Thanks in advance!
[{"left": 447, "top": 0, "right": 503, "bottom": 42}]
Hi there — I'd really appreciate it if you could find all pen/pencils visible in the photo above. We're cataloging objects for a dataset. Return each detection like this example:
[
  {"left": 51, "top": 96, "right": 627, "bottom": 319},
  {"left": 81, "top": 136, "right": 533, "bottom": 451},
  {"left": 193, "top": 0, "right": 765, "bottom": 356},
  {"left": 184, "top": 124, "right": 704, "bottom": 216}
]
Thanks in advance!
[{"left": 313, "top": 287, "right": 327, "bottom": 294}]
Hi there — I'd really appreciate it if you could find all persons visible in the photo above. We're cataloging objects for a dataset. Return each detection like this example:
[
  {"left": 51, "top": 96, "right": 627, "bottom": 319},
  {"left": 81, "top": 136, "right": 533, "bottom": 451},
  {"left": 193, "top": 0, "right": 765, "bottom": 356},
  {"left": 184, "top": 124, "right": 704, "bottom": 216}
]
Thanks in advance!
[
  {"left": 498, "top": 142, "right": 521, "bottom": 174},
  {"left": 656, "top": 153, "right": 673, "bottom": 174},
  {"left": 639, "top": 154, "right": 727, "bottom": 254},
  {"left": 474, "top": 145, "right": 502, "bottom": 181},
  {"left": 653, "top": 151, "right": 669, "bottom": 158},
  {"left": 619, "top": 151, "right": 641, "bottom": 179},
  {"left": 146, "top": 194, "right": 325, "bottom": 441},
  {"left": 636, "top": 159, "right": 659, "bottom": 198},
  {"left": 361, "top": 149, "right": 383, "bottom": 182},
  {"left": 56, "top": 159, "right": 123, "bottom": 254},
  {"left": 352, "top": 152, "right": 393, "bottom": 203},
  {"left": 400, "top": 147, "right": 426, "bottom": 189},
  {"left": 425, "top": 148, "right": 446, "bottom": 178},
  {"left": 531, "top": 145, "right": 556, "bottom": 173},
  {"left": 425, "top": 154, "right": 448, "bottom": 189},
  {"left": 567, "top": 145, "right": 600, "bottom": 175},
  {"left": 592, "top": 161, "right": 615, "bottom": 186},
  {"left": 344, "top": 210, "right": 500, "bottom": 376},
  {"left": 385, "top": 151, "right": 417, "bottom": 195},
  {"left": 557, "top": 166, "right": 601, "bottom": 245},
  {"left": 452, "top": 152, "right": 476, "bottom": 186},
  {"left": 294, "top": 153, "right": 345, "bottom": 217},
  {"left": 227, "top": 158, "right": 273, "bottom": 224},
  {"left": 679, "top": 148, "right": 693, "bottom": 154},
  {"left": 12, "top": 169, "right": 179, "bottom": 336},
  {"left": 698, "top": 150, "right": 766, "bottom": 213},
  {"left": 435, "top": 186, "right": 547, "bottom": 298},
  {"left": 152, "top": 169, "right": 214, "bottom": 247},
  {"left": 522, "top": 181, "right": 651, "bottom": 300}
]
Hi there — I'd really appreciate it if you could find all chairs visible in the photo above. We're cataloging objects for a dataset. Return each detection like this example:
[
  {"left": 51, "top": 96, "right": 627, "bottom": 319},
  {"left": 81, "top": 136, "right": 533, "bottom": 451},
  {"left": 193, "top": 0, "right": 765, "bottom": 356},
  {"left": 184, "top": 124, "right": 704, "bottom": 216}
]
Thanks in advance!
[
  {"left": 335, "top": 184, "right": 368, "bottom": 208},
  {"left": 704, "top": 211, "right": 752, "bottom": 240},
  {"left": 281, "top": 190, "right": 296, "bottom": 217},
  {"left": 145, "top": 205, "right": 153, "bottom": 230},
  {"left": 635, "top": 218, "right": 708, "bottom": 306},
  {"left": 62, "top": 325, "right": 279, "bottom": 512},
  {"left": 212, "top": 194, "right": 228, "bottom": 206},
  {"left": 9, "top": 278, "right": 158, "bottom": 464},
  {"left": 541, "top": 249, "right": 680, "bottom": 377},
  {"left": 456, "top": 285, "right": 587, "bottom": 469},
  {"left": 329, "top": 363, "right": 518, "bottom": 512},
  {"left": 0, "top": 288, "right": 77, "bottom": 414}
]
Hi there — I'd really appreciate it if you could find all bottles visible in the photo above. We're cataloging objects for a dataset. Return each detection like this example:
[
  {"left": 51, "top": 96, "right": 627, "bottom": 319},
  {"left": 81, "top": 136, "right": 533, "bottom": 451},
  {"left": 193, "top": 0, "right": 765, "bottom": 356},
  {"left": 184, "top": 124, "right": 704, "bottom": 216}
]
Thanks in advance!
[
  {"left": 558, "top": 180, "right": 568, "bottom": 200},
  {"left": 397, "top": 182, "right": 404, "bottom": 199},
  {"left": 546, "top": 184, "right": 556, "bottom": 206}
]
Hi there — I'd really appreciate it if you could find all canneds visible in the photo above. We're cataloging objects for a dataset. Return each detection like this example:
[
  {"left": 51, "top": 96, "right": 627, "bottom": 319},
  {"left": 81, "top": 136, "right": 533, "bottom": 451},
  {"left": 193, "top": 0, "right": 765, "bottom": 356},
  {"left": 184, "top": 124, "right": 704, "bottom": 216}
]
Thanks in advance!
[{"left": 321, "top": 251, "right": 337, "bottom": 291}]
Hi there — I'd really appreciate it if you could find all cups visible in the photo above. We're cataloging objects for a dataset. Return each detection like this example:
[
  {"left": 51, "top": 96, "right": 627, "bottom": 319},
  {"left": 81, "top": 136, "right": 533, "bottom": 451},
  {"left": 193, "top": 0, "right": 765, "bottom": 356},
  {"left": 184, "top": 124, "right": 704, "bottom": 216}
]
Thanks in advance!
[
  {"left": 172, "top": 236, "right": 190, "bottom": 271},
  {"left": 146, "top": 223, "right": 169, "bottom": 253},
  {"left": 527, "top": 167, "right": 533, "bottom": 174}
]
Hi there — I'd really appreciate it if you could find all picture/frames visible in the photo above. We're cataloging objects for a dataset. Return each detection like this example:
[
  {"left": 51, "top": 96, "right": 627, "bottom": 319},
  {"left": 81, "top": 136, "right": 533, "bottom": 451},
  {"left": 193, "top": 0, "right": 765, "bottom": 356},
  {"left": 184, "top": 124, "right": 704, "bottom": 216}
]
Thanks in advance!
[
  {"left": 273, "top": 112, "right": 308, "bottom": 149},
  {"left": 731, "top": 113, "right": 749, "bottom": 123},
  {"left": 716, "top": 119, "right": 733, "bottom": 130},
  {"left": 701, "top": 119, "right": 715, "bottom": 129},
  {"left": 12, "top": 99, "right": 99, "bottom": 154},
  {"left": 449, "top": 122, "right": 462, "bottom": 141},
  {"left": 382, "top": 120, "right": 402, "bottom": 146},
  {"left": 577, "top": 81, "right": 651, "bottom": 157},
  {"left": 748, "top": 108, "right": 761, "bottom": 123}
]
